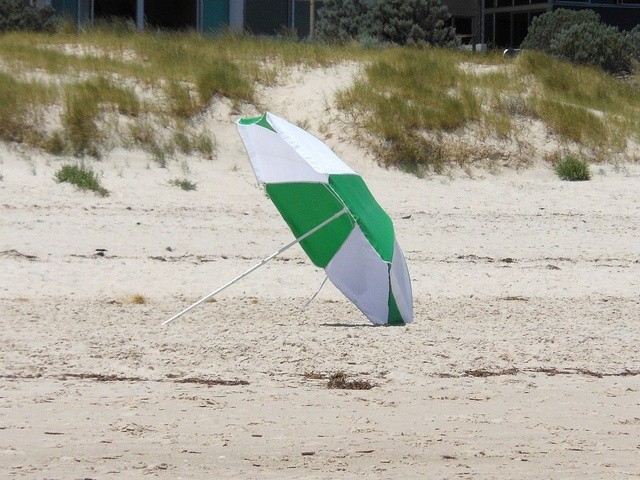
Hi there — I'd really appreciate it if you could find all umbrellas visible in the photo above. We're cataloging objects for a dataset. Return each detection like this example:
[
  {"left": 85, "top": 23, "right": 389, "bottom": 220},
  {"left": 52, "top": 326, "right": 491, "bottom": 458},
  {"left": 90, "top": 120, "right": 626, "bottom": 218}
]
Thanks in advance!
[{"left": 163, "top": 111, "right": 412, "bottom": 326}]
